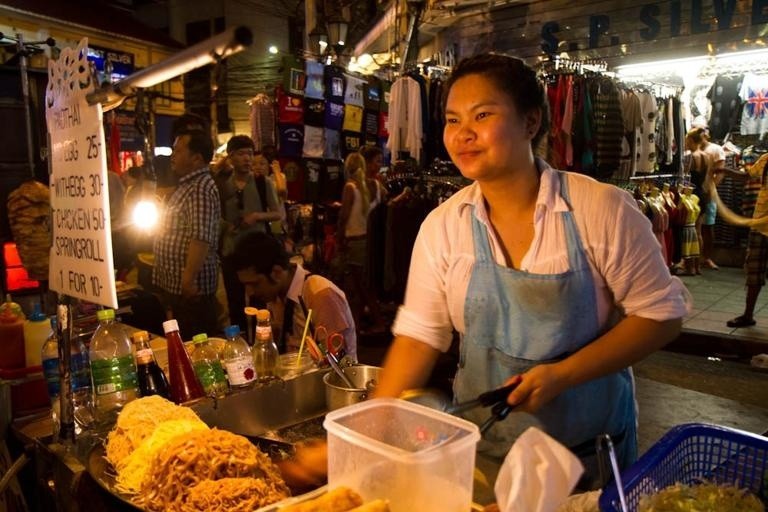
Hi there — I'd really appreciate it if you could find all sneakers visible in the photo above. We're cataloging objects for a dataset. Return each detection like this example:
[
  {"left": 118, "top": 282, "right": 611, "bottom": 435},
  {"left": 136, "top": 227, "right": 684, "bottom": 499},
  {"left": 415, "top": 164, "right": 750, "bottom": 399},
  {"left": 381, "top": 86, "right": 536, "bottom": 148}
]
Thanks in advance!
[{"left": 727, "top": 315, "right": 756, "bottom": 327}]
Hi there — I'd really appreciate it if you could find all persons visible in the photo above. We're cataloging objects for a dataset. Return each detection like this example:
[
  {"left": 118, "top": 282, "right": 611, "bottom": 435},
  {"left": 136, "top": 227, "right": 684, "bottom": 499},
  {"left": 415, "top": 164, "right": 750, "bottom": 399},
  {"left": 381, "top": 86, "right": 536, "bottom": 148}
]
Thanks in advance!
[
  {"left": 633, "top": 183, "right": 702, "bottom": 266},
  {"left": 712, "top": 134, "right": 767, "bottom": 327},
  {"left": 674, "top": 128, "right": 727, "bottom": 270},
  {"left": 283, "top": 52, "right": 693, "bottom": 493},
  {"left": 684, "top": 131, "right": 710, "bottom": 276}
]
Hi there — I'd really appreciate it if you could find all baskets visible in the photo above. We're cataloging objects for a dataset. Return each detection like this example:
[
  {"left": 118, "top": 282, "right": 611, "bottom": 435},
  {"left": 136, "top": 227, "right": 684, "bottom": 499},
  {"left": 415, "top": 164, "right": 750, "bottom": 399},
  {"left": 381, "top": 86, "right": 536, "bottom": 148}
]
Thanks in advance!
[{"left": 599, "top": 422, "right": 768, "bottom": 510}]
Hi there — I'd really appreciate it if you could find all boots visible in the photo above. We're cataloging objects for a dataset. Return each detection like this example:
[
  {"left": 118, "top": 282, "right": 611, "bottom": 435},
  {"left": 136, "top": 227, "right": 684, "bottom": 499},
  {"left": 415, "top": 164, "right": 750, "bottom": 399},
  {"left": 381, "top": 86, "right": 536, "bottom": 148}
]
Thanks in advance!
[{"left": 677, "top": 255, "right": 720, "bottom": 276}]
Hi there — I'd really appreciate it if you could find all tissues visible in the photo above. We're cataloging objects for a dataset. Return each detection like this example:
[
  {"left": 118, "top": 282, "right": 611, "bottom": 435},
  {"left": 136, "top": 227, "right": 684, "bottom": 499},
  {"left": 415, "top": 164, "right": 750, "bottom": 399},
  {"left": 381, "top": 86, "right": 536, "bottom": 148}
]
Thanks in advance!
[{"left": 483, "top": 427, "right": 585, "bottom": 512}]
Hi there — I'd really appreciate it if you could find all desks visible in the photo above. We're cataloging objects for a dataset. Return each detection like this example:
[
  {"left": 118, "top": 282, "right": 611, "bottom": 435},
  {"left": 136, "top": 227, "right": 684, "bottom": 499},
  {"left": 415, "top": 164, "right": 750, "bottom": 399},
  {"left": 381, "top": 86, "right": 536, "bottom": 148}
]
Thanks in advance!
[{"left": 0, "top": 315, "right": 184, "bottom": 512}]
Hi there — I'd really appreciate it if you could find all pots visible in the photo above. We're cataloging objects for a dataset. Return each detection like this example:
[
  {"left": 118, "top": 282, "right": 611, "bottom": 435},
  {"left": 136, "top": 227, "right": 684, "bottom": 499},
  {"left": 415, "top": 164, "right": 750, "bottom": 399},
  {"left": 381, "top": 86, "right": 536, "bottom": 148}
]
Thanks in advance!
[{"left": 321, "top": 365, "right": 387, "bottom": 413}]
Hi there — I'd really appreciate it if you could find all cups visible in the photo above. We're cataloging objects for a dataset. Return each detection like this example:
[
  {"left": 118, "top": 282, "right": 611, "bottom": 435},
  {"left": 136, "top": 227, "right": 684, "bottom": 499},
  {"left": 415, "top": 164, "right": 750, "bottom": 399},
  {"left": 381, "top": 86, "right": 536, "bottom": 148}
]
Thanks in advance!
[{"left": 276, "top": 352, "right": 314, "bottom": 380}]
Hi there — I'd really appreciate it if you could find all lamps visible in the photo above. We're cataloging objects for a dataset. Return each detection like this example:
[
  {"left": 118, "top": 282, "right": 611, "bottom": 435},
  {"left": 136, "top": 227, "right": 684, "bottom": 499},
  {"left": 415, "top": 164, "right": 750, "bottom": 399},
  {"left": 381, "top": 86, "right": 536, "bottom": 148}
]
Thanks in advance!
[{"left": 309, "top": 24, "right": 347, "bottom": 65}]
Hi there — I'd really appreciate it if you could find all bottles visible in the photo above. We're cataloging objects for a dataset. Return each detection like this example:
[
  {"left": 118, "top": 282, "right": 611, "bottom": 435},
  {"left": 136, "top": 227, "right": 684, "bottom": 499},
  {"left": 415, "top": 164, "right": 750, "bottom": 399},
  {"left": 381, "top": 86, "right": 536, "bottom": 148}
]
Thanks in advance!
[
  {"left": 129, "top": 324, "right": 175, "bottom": 409},
  {"left": 38, "top": 310, "right": 96, "bottom": 439},
  {"left": 188, "top": 333, "right": 226, "bottom": 400},
  {"left": 84, "top": 310, "right": 139, "bottom": 427},
  {"left": 216, "top": 326, "right": 258, "bottom": 392},
  {"left": 0, "top": 303, "right": 29, "bottom": 382},
  {"left": 159, "top": 319, "right": 205, "bottom": 407},
  {"left": 244, "top": 306, "right": 281, "bottom": 386}
]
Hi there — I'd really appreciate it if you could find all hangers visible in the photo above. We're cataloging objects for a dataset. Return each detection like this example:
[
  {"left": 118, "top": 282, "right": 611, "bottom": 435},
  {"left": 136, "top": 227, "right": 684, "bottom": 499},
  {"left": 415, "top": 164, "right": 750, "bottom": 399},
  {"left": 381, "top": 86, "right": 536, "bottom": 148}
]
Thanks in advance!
[{"left": 542, "top": 56, "right": 606, "bottom": 80}]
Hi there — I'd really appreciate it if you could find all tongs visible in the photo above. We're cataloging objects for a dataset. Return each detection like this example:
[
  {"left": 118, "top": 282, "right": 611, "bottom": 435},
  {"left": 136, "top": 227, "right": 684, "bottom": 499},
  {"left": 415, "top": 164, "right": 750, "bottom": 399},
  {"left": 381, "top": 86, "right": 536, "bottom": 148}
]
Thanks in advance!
[
  {"left": 325, "top": 351, "right": 356, "bottom": 389},
  {"left": 594, "top": 434, "right": 627, "bottom": 512}
]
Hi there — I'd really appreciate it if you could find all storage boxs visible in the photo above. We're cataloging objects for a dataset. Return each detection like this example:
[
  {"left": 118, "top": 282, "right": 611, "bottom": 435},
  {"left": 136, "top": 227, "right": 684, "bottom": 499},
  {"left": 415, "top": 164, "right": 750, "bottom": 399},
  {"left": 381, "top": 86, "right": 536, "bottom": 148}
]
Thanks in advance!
[{"left": 322, "top": 397, "right": 482, "bottom": 512}]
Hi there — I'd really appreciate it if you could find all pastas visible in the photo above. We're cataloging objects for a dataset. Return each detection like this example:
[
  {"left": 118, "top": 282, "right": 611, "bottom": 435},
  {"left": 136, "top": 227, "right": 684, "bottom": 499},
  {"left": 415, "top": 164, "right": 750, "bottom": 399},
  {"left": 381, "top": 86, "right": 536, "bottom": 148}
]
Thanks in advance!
[{"left": 99, "top": 394, "right": 292, "bottom": 512}]
